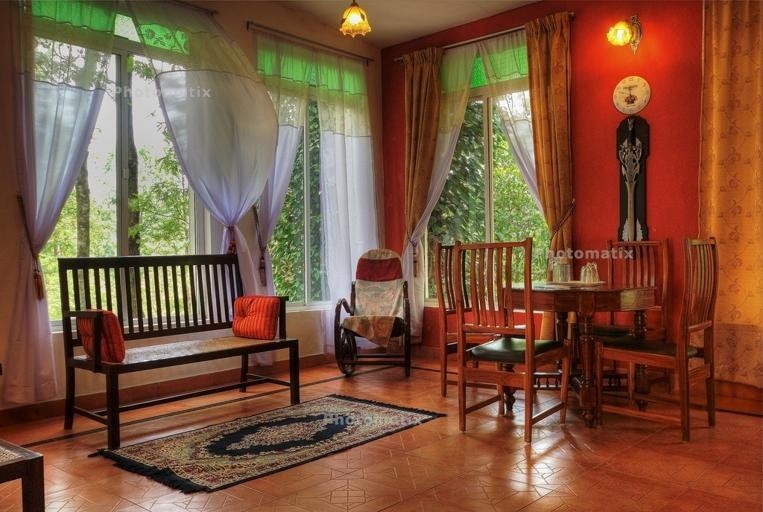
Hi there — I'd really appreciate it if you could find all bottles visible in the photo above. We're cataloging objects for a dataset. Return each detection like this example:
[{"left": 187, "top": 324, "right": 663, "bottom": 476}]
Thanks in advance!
[{"left": 550, "top": 257, "right": 570, "bottom": 283}]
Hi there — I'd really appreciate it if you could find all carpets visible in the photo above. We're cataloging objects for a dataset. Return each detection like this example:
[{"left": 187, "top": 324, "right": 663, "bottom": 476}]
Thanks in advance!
[{"left": 88, "top": 393, "right": 447, "bottom": 494}]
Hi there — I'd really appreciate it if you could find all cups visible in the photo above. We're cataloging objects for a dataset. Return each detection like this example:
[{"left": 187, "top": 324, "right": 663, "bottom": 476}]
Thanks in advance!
[{"left": 579, "top": 263, "right": 600, "bottom": 282}]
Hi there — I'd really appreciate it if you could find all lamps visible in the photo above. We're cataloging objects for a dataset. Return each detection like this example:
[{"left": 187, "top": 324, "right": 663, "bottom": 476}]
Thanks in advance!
[
  {"left": 339, "top": 0, "right": 373, "bottom": 41},
  {"left": 607, "top": 15, "right": 643, "bottom": 55}
]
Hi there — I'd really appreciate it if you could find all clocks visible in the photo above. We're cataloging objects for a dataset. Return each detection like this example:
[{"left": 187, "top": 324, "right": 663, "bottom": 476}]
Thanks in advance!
[{"left": 609, "top": 70, "right": 653, "bottom": 117}]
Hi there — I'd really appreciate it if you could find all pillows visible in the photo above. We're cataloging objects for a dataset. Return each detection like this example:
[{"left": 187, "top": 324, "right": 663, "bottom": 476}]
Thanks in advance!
[
  {"left": 77, "top": 309, "right": 125, "bottom": 363},
  {"left": 231, "top": 296, "right": 281, "bottom": 340}
]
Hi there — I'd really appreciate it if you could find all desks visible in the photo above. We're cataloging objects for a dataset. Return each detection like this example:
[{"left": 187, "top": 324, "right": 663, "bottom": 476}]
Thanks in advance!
[{"left": 0, "top": 438, "right": 45, "bottom": 512}]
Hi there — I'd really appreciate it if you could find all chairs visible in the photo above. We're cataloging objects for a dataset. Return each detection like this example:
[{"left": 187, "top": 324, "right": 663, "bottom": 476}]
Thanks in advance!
[
  {"left": 334, "top": 248, "right": 411, "bottom": 377},
  {"left": 58, "top": 254, "right": 300, "bottom": 449}
]
